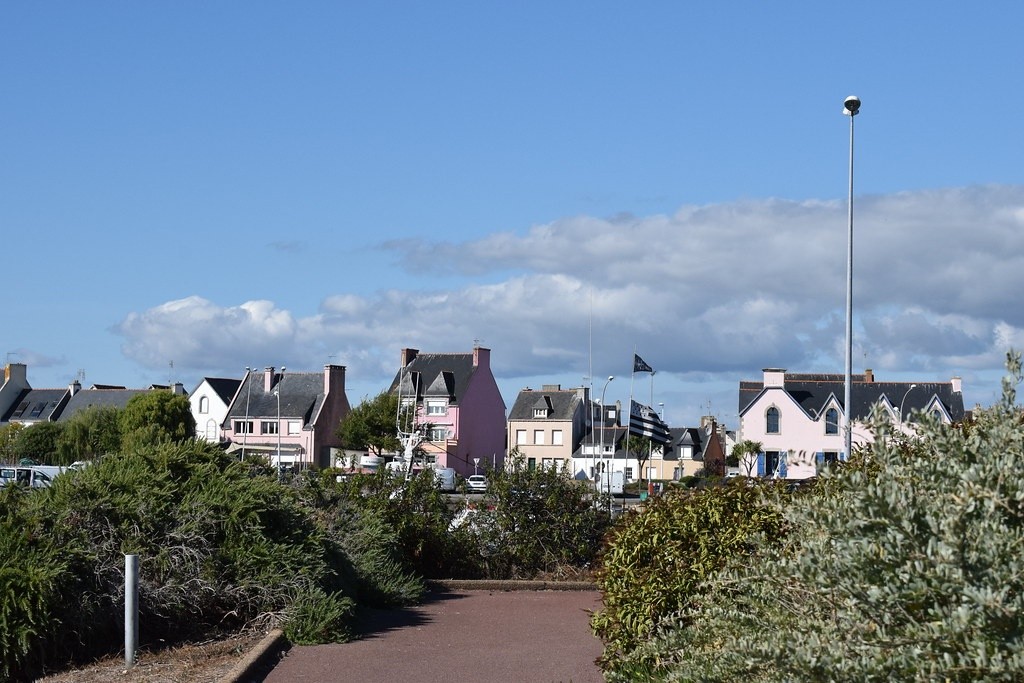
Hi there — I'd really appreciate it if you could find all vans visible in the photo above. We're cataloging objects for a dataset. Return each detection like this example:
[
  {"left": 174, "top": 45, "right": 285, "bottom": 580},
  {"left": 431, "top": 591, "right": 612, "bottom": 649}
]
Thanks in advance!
[
  {"left": 433, "top": 468, "right": 457, "bottom": 494},
  {"left": 0, "top": 468, "right": 53, "bottom": 490}
]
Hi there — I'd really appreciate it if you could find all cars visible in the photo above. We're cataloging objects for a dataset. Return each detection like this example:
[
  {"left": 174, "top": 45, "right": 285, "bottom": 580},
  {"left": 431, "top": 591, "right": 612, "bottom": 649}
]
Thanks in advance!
[{"left": 466, "top": 475, "right": 490, "bottom": 494}]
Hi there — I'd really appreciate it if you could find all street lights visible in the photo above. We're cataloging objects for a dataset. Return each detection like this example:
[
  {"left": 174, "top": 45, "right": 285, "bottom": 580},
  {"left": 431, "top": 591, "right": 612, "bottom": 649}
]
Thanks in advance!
[
  {"left": 272, "top": 366, "right": 286, "bottom": 485},
  {"left": 240, "top": 366, "right": 258, "bottom": 463},
  {"left": 838, "top": 95, "right": 862, "bottom": 461},
  {"left": 893, "top": 384, "right": 917, "bottom": 434},
  {"left": 647, "top": 370, "right": 657, "bottom": 496},
  {"left": 658, "top": 402, "right": 665, "bottom": 494},
  {"left": 599, "top": 375, "right": 615, "bottom": 493}
]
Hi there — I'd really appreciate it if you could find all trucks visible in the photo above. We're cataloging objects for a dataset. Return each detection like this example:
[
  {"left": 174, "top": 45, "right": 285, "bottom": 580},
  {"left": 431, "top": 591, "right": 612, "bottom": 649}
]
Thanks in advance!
[{"left": 28, "top": 466, "right": 78, "bottom": 484}]
